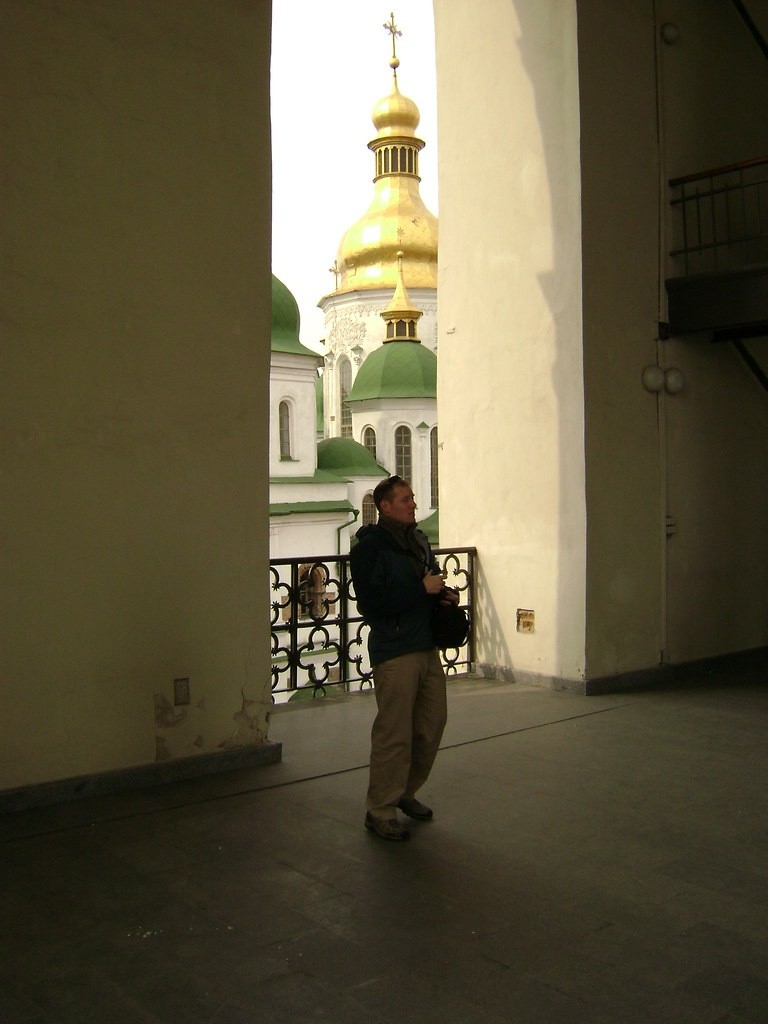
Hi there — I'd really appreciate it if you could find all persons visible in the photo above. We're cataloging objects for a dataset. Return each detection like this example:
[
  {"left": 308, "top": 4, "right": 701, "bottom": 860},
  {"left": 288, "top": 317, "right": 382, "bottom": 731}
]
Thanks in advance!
[{"left": 348, "top": 475, "right": 461, "bottom": 841}]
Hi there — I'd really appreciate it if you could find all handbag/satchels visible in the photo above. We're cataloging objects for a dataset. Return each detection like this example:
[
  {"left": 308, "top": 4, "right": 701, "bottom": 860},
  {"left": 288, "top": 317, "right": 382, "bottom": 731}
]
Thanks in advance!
[{"left": 432, "top": 591, "right": 468, "bottom": 648}]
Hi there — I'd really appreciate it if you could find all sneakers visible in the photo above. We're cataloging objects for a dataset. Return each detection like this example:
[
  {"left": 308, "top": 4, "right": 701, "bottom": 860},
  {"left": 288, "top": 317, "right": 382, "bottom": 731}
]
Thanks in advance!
[
  {"left": 365, "top": 810, "right": 410, "bottom": 841},
  {"left": 396, "top": 797, "right": 433, "bottom": 820}
]
